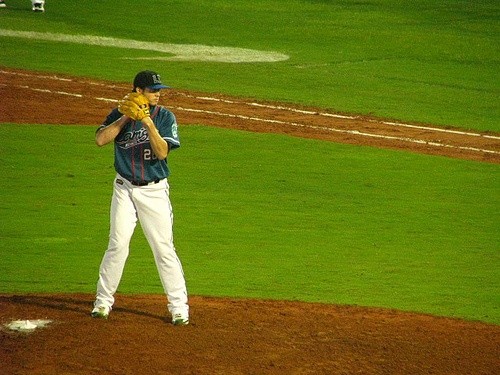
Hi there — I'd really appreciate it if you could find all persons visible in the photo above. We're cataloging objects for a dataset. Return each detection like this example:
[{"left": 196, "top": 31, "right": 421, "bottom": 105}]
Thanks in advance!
[{"left": 91, "top": 69, "right": 190, "bottom": 326}]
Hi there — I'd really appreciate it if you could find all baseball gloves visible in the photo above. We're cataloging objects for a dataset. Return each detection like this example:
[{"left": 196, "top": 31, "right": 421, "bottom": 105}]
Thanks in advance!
[{"left": 116, "top": 93, "right": 152, "bottom": 123}]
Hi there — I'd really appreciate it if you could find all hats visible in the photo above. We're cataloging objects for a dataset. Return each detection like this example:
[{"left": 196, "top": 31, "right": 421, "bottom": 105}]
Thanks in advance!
[{"left": 134, "top": 70, "right": 168, "bottom": 89}]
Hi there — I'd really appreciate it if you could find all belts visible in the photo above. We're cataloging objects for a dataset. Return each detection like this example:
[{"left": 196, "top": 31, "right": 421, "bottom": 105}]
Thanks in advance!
[{"left": 120, "top": 175, "right": 166, "bottom": 186}]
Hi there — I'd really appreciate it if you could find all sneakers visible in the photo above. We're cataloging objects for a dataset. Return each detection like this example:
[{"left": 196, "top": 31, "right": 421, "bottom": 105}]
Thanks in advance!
[
  {"left": 172, "top": 310, "right": 189, "bottom": 325},
  {"left": 91, "top": 307, "right": 108, "bottom": 319}
]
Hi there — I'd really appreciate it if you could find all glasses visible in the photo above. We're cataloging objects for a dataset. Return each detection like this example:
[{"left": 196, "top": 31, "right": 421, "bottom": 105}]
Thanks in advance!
[{"left": 149, "top": 88, "right": 160, "bottom": 93}]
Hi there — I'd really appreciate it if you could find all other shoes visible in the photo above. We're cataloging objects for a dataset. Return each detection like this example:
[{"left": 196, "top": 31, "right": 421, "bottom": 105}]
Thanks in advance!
[
  {"left": 0, "top": 3, "right": 6, "bottom": 7},
  {"left": 32, "top": 2, "right": 44, "bottom": 12}
]
